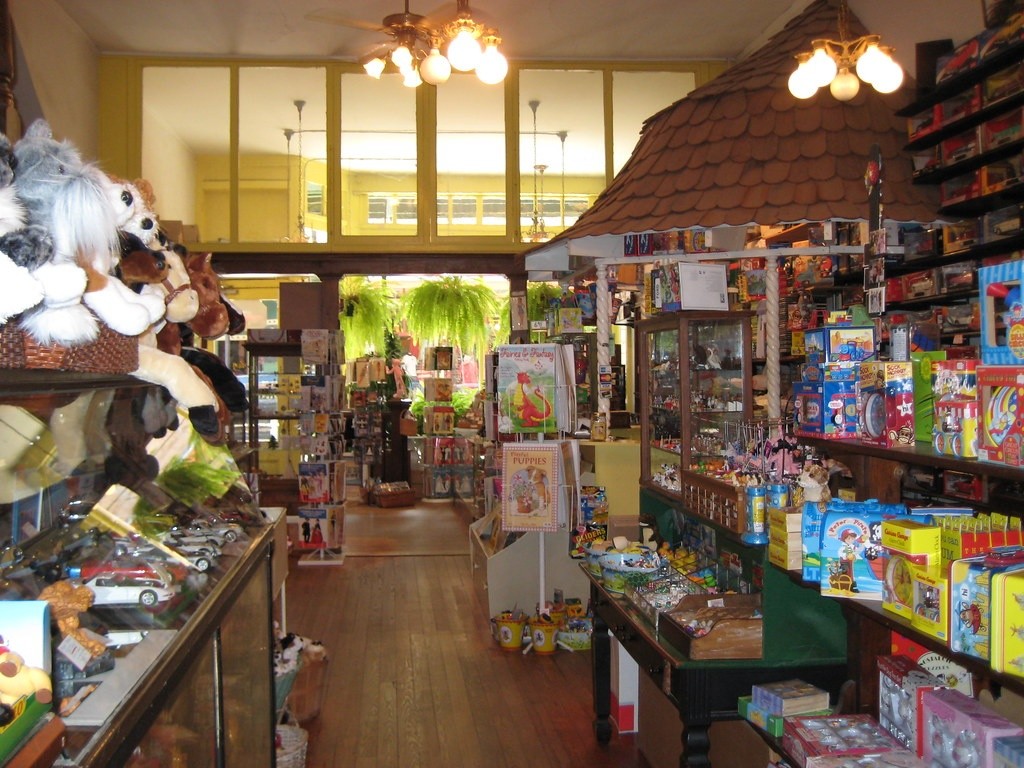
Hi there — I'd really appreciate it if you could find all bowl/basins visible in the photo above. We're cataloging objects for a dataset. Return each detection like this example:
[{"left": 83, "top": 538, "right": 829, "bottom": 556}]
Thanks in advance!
[
  {"left": 597, "top": 554, "right": 664, "bottom": 594},
  {"left": 583, "top": 542, "right": 626, "bottom": 578}
]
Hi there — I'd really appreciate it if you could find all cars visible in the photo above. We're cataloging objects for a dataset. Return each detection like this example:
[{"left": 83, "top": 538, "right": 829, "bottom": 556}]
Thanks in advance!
[{"left": 64, "top": 512, "right": 252, "bottom": 608}]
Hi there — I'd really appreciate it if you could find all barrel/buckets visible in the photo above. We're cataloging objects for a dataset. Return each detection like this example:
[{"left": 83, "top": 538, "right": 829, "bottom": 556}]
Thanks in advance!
[
  {"left": 527, "top": 617, "right": 561, "bottom": 655},
  {"left": 491, "top": 612, "right": 529, "bottom": 651}
]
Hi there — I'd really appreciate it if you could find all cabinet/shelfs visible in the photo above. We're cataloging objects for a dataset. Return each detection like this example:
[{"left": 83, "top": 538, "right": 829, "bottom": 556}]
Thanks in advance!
[
  {"left": 244, "top": 328, "right": 348, "bottom": 566},
  {"left": 835, "top": 40, "right": 1024, "bottom": 346},
  {"left": 469, "top": 500, "right": 590, "bottom": 637},
  {"left": 0, "top": 369, "right": 276, "bottom": 768},
  {"left": 452, "top": 427, "right": 486, "bottom": 522},
  {"left": 631, "top": 309, "right": 758, "bottom": 501},
  {"left": 744, "top": 435, "right": 1024, "bottom": 768},
  {"left": 380, "top": 398, "right": 413, "bottom": 487},
  {"left": 421, "top": 346, "right": 459, "bottom": 504}
]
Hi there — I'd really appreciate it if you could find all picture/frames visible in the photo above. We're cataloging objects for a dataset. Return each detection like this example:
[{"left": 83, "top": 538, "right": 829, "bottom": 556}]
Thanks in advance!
[{"left": 501, "top": 443, "right": 559, "bottom": 533}]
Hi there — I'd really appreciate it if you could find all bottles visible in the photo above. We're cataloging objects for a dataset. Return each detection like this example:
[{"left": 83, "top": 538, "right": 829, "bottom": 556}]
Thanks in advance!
[{"left": 591, "top": 412, "right": 607, "bottom": 441}]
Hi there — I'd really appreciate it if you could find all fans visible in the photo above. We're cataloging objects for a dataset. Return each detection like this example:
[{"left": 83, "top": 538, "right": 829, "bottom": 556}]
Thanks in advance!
[{"left": 304, "top": 0, "right": 503, "bottom": 63}]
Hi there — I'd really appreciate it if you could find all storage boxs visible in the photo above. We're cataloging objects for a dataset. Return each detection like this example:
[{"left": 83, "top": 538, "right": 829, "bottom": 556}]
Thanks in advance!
[
  {"left": 277, "top": 282, "right": 339, "bottom": 330},
  {"left": 657, "top": 593, "right": 761, "bottom": 659},
  {"left": 735, "top": 0, "right": 1024, "bottom": 768},
  {"left": 677, "top": 466, "right": 746, "bottom": 533}
]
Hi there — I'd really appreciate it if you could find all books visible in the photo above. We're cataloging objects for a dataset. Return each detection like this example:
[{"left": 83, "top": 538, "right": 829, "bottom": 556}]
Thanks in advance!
[{"left": 297, "top": 329, "right": 348, "bottom": 549}]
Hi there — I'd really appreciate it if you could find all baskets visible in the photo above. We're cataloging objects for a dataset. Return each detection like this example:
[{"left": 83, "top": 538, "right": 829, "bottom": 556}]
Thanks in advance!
[
  {"left": 399, "top": 409, "right": 417, "bottom": 436},
  {"left": 275, "top": 724, "right": 308, "bottom": 768},
  {"left": 0, "top": 319, "right": 139, "bottom": 373},
  {"left": 373, "top": 488, "right": 417, "bottom": 507}
]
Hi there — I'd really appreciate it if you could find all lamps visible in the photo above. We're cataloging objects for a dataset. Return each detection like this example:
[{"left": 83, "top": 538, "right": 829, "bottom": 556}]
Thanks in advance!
[
  {"left": 522, "top": 100, "right": 557, "bottom": 242},
  {"left": 358, "top": 41, "right": 427, "bottom": 88},
  {"left": 421, "top": 0, "right": 509, "bottom": 86}
]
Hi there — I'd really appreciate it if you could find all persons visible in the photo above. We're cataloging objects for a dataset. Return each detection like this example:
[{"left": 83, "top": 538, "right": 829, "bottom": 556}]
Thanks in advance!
[
  {"left": 302, "top": 516, "right": 312, "bottom": 544},
  {"left": 313, "top": 518, "right": 322, "bottom": 544}
]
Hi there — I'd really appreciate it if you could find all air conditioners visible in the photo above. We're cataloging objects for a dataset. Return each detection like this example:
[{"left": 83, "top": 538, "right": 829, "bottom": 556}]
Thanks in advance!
[{"left": 788, "top": 0, "right": 904, "bottom": 101}]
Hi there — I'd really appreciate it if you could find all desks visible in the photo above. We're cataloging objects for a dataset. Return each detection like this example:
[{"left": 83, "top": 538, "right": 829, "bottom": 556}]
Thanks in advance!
[
  {"left": 580, "top": 477, "right": 862, "bottom": 768},
  {"left": 576, "top": 440, "right": 640, "bottom": 515}
]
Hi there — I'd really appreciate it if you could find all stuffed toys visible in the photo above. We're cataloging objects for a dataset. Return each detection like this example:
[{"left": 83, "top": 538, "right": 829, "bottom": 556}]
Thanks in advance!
[
  {"left": 271, "top": 632, "right": 326, "bottom": 677},
  {"left": 636, "top": 513, "right": 664, "bottom": 552},
  {"left": 0, "top": 118, "right": 249, "bottom": 441},
  {"left": 796, "top": 465, "right": 832, "bottom": 515},
  {"left": 0, "top": 646, "right": 53, "bottom": 709}
]
